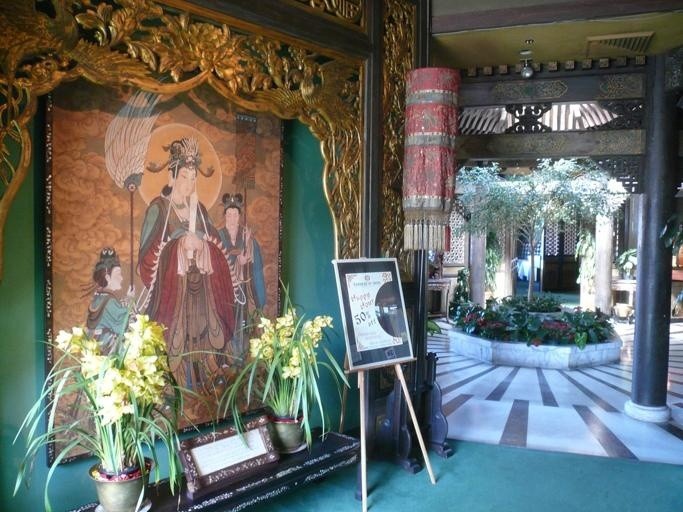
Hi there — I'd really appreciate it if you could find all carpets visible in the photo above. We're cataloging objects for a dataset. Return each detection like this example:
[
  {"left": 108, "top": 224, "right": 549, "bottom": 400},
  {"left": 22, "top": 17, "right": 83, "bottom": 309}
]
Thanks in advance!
[{"left": 245, "top": 434, "right": 682, "bottom": 511}]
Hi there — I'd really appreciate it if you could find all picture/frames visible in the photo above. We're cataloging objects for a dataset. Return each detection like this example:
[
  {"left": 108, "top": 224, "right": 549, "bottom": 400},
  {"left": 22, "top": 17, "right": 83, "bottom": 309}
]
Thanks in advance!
[{"left": 331, "top": 255, "right": 416, "bottom": 372}]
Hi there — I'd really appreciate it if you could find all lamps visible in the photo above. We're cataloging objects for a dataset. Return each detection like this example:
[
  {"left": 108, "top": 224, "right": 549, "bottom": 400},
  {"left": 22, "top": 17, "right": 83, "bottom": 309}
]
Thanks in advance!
[{"left": 514, "top": 47, "right": 539, "bottom": 78}]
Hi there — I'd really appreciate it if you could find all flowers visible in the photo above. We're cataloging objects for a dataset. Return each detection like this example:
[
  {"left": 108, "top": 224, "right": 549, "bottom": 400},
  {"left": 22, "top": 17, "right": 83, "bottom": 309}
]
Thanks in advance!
[
  {"left": 207, "top": 292, "right": 351, "bottom": 458},
  {"left": 6, "top": 290, "right": 201, "bottom": 511}
]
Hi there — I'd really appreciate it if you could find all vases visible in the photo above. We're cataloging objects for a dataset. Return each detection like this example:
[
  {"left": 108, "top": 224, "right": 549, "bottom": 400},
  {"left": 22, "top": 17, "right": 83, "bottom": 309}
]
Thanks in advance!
[
  {"left": 91, "top": 454, "right": 153, "bottom": 511},
  {"left": 269, "top": 411, "right": 309, "bottom": 457}
]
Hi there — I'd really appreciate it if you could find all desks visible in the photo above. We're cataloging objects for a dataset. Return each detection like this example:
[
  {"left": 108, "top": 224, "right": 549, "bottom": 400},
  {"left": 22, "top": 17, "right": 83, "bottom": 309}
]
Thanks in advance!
[{"left": 610, "top": 280, "right": 636, "bottom": 322}]
